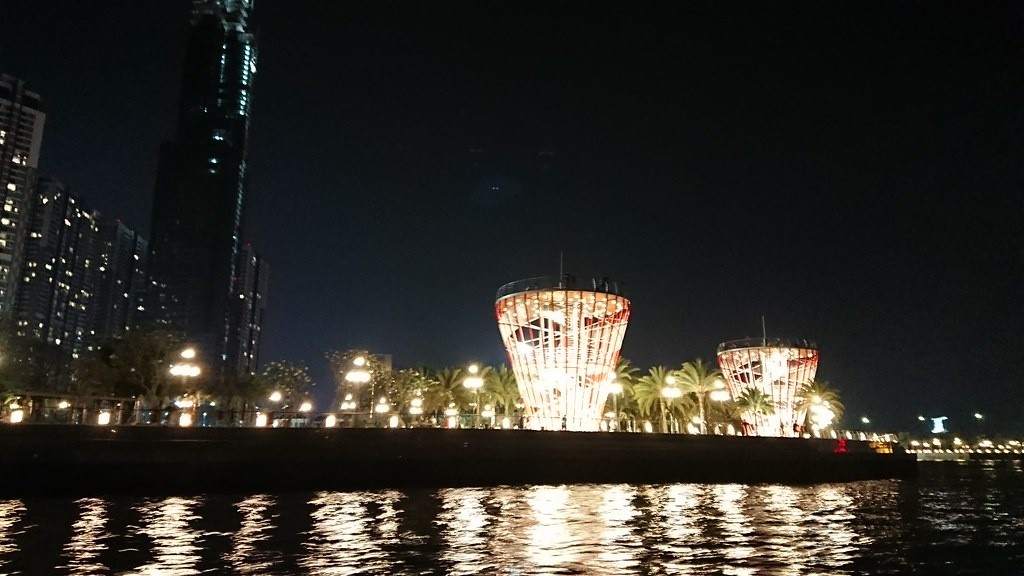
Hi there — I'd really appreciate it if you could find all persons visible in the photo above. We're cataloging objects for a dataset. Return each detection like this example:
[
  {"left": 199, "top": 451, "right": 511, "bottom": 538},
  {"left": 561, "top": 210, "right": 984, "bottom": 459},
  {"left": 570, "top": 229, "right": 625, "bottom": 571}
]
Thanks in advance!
[
  {"left": 620, "top": 417, "right": 627, "bottom": 431},
  {"left": 511, "top": 409, "right": 521, "bottom": 429},
  {"left": 792, "top": 420, "right": 801, "bottom": 437},
  {"left": 561, "top": 415, "right": 567, "bottom": 430},
  {"left": 741, "top": 418, "right": 750, "bottom": 436}
]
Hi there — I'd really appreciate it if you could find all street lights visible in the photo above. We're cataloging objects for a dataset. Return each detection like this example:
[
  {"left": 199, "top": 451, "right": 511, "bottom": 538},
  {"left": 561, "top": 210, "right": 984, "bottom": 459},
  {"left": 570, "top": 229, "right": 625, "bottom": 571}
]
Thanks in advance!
[
  {"left": 813, "top": 397, "right": 832, "bottom": 439},
  {"left": 172, "top": 346, "right": 201, "bottom": 425},
  {"left": 610, "top": 375, "right": 624, "bottom": 432},
  {"left": 661, "top": 377, "right": 681, "bottom": 433},
  {"left": 344, "top": 356, "right": 371, "bottom": 427},
  {"left": 709, "top": 379, "right": 731, "bottom": 436},
  {"left": 463, "top": 366, "right": 482, "bottom": 427}
]
